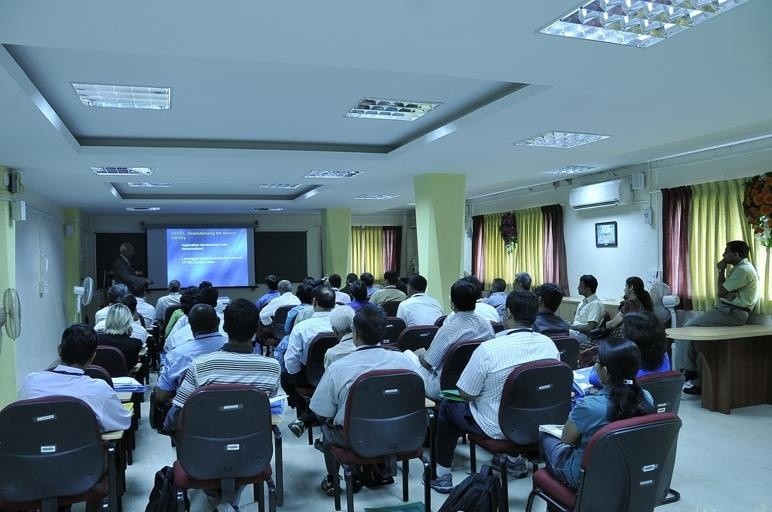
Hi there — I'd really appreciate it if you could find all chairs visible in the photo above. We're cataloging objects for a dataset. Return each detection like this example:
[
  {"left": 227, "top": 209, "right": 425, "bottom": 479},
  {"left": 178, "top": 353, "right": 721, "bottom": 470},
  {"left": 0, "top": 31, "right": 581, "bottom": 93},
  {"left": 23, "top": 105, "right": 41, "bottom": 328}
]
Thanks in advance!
[
  {"left": 385, "top": 325, "right": 439, "bottom": 352},
  {"left": 90, "top": 345, "right": 142, "bottom": 432},
  {"left": 525, "top": 412, "right": 683, "bottom": 512},
  {"left": 381, "top": 300, "right": 401, "bottom": 317},
  {"left": 260, "top": 304, "right": 298, "bottom": 357},
  {"left": 467, "top": 360, "right": 574, "bottom": 512},
  {"left": 575, "top": 313, "right": 613, "bottom": 368},
  {"left": 434, "top": 315, "right": 447, "bottom": 327},
  {"left": 164, "top": 304, "right": 182, "bottom": 324},
  {"left": 0, "top": 395, "right": 125, "bottom": 512},
  {"left": 386, "top": 317, "right": 406, "bottom": 342},
  {"left": 173, "top": 385, "right": 283, "bottom": 512},
  {"left": 635, "top": 370, "right": 685, "bottom": 415},
  {"left": 439, "top": 340, "right": 480, "bottom": 390},
  {"left": 294, "top": 332, "right": 339, "bottom": 445},
  {"left": 549, "top": 335, "right": 579, "bottom": 370},
  {"left": 329, "top": 369, "right": 431, "bottom": 512},
  {"left": 44, "top": 365, "right": 133, "bottom": 466}
]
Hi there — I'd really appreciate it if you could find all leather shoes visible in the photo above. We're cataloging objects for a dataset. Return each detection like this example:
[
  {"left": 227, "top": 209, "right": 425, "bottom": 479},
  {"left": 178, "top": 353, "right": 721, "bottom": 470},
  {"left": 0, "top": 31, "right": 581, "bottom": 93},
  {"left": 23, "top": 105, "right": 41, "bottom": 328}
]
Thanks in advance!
[
  {"left": 684, "top": 385, "right": 701, "bottom": 396},
  {"left": 682, "top": 369, "right": 698, "bottom": 382}
]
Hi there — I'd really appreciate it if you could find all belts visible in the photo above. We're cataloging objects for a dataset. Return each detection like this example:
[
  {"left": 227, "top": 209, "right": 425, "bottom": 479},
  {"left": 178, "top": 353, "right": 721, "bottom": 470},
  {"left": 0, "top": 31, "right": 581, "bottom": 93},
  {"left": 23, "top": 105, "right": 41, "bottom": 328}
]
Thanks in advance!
[{"left": 722, "top": 301, "right": 750, "bottom": 312}]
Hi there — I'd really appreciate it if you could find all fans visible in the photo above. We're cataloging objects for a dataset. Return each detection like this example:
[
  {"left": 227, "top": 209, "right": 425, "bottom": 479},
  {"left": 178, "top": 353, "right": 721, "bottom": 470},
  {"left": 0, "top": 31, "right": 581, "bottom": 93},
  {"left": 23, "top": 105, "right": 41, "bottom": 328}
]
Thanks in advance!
[
  {"left": 72, "top": 277, "right": 94, "bottom": 324},
  {"left": 0, "top": 287, "right": 22, "bottom": 354},
  {"left": 650, "top": 283, "right": 680, "bottom": 370}
]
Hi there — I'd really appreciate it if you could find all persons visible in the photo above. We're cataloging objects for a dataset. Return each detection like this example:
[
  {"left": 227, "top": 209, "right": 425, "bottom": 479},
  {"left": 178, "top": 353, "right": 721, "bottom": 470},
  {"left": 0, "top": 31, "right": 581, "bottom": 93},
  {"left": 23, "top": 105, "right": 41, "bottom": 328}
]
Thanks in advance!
[
  {"left": 308, "top": 304, "right": 426, "bottom": 497},
  {"left": 368, "top": 270, "right": 407, "bottom": 306},
  {"left": 94, "top": 284, "right": 128, "bottom": 324},
  {"left": 155, "top": 280, "right": 182, "bottom": 321},
  {"left": 283, "top": 285, "right": 337, "bottom": 408},
  {"left": 167, "top": 286, "right": 224, "bottom": 346},
  {"left": 129, "top": 280, "right": 155, "bottom": 328},
  {"left": 564, "top": 274, "right": 605, "bottom": 344},
  {"left": 17, "top": 324, "right": 132, "bottom": 512},
  {"left": 199, "top": 281, "right": 223, "bottom": 313},
  {"left": 512, "top": 272, "right": 532, "bottom": 290},
  {"left": 674, "top": 240, "right": 760, "bottom": 396},
  {"left": 256, "top": 275, "right": 280, "bottom": 311},
  {"left": 162, "top": 298, "right": 281, "bottom": 512},
  {"left": 345, "top": 279, "right": 378, "bottom": 313},
  {"left": 92, "top": 294, "right": 147, "bottom": 345},
  {"left": 580, "top": 276, "right": 655, "bottom": 366},
  {"left": 571, "top": 309, "right": 671, "bottom": 412},
  {"left": 340, "top": 273, "right": 358, "bottom": 302},
  {"left": 328, "top": 274, "right": 351, "bottom": 305},
  {"left": 256, "top": 280, "right": 302, "bottom": 345},
  {"left": 285, "top": 283, "right": 315, "bottom": 334},
  {"left": 414, "top": 280, "right": 496, "bottom": 400},
  {"left": 164, "top": 308, "right": 184, "bottom": 339},
  {"left": 286, "top": 305, "right": 357, "bottom": 438},
  {"left": 360, "top": 273, "right": 380, "bottom": 297},
  {"left": 96, "top": 303, "right": 143, "bottom": 375},
  {"left": 163, "top": 287, "right": 227, "bottom": 351},
  {"left": 154, "top": 303, "right": 227, "bottom": 404},
  {"left": 323, "top": 305, "right": 355, "bottom": 372},
  {"left": 538, "top": 337, "right": 659, "bottom": 512},
  {"left": 477, "top": 278, "right": 508, "bottom": 320},
  {"left": 396, "top": 275, "right": 444, "bottom": 328},
  {"left": 532, "top": 282, "right": 569, "bottom": 337},
  {"left": 442, "top": 276, "right": 500, "bottom": 324},
  {"left": 113, "top": 242, "right": 152, "bottom": 298},
  {"left": 421, "top": 289, "right": 561, "bottom": 493}
]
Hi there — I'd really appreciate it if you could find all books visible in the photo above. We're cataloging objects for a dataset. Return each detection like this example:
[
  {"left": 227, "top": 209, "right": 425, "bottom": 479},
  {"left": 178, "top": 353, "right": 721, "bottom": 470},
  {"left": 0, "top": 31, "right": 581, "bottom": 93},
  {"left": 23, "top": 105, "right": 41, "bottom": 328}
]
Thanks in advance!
[
  {"left": 538, "top": 424, "right": 565, "bottom": 439},
  {"left": 572, "top": 367, "right": 598, "bottom": 396},
  {"left": 268, "top": 394, "right": 289, "bottom": 414},
  {"left": 112, "top": 377, "right": 145, "bottom": 393}
]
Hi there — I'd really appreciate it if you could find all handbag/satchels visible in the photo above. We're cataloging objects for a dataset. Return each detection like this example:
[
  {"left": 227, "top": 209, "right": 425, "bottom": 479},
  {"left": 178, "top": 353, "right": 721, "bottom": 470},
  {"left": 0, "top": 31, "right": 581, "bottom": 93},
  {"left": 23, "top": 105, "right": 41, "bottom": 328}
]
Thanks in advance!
[{"left": 591, "top": 327, "right": 611, "bottom": 338}]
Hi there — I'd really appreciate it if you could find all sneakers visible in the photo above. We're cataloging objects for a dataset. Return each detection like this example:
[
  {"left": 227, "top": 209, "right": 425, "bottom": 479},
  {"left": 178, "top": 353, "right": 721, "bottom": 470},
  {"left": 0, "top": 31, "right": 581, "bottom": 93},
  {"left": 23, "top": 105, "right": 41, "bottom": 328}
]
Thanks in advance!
[
  {"left": 321, "top": 475, "right": 335, "bottom": 496},
  {"left": 421, "top": 473, "right": 453, "bottom": 493},
  {"left": 98, "top": 498, "right": 111, "bottom": 512},
  {"left": 287, "top": 416, "right": 306, "bottom": 438},
  {"left": 491, "top": 455, "right": 528, "bottom": 479}
]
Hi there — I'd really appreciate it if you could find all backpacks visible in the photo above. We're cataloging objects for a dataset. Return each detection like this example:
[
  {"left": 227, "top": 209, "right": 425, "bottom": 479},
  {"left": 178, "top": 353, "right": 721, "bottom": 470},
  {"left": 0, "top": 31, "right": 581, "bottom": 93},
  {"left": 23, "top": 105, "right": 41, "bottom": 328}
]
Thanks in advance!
[
  {"left": 436, "top": 463, "right": 506, "bottom": 512},
  {"left": 145, "top": 465, "right": 192, "bottom": 512}
]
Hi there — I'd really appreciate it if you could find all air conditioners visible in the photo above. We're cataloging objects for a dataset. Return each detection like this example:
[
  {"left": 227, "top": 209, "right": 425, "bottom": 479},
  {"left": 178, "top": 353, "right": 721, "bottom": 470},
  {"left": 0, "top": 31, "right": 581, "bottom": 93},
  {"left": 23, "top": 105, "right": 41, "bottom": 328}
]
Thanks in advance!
[{"left": 567, "top": 176, "right": 633, "bottom": 213}]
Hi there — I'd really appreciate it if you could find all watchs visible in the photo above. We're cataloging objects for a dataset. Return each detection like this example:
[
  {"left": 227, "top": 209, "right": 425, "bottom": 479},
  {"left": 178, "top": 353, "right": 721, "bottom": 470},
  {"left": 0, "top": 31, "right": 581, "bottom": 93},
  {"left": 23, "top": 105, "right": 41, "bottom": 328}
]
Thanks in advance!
[{"left": 717, "top": 269, "right": 724, "bottom": 273}]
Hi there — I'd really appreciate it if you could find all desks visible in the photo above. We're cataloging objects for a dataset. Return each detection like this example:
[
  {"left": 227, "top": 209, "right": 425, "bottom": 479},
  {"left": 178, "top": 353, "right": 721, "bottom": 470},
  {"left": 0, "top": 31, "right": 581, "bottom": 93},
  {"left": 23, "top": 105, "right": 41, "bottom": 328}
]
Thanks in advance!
[{"left": 665, "top": 323, "right": 772, "bottom": 414}]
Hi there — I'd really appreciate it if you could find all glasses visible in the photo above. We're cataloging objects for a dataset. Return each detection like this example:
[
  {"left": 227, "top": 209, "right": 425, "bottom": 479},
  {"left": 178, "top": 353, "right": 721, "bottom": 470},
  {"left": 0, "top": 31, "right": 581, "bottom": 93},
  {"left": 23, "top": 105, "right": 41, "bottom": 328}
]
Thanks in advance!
[
  {"left": 350, "top": 324, "right": 354, "bottom": 330},
  {"left": 592, "top": 356, "right": 602, "bottom": 365},
  {"left": 501, "top": 308, "right": 507, "bottom": 312}
]
